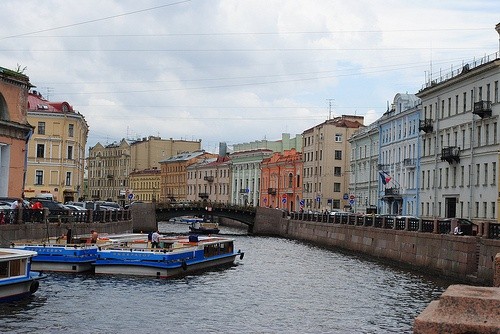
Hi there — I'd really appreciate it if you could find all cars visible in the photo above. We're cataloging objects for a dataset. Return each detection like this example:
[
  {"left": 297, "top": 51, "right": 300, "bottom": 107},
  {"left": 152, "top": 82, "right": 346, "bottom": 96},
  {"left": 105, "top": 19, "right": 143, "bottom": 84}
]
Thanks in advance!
[
  {"left": 331, "top": 211, "right": 478, "bottom": 236},
  {"left": 0, "top": 196, "right": 144, "bottom": 222}
]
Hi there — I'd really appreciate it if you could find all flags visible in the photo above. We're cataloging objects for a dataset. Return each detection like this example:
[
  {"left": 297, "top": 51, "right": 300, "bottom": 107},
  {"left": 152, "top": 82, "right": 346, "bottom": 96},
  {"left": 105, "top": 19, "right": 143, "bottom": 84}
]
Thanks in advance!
[
  {"left": 379, "top": 173, "right": 386, "bottom": 185},
  {"left": 383, "top": 172, "right": 392, "bottom": 183}
]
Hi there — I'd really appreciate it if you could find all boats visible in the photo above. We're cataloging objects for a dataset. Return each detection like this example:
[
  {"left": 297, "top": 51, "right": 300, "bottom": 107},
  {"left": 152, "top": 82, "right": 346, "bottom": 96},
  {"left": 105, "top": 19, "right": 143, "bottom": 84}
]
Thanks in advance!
[
  {"left": 11, "top": 239, "right": 99, "bottom": 273},
  {"left": 91, "top": 236, "right": 246, "bottom": 278},
  {"left": 188, "top": 220, "right": 221, "bottom": 233},
  {"left": 0, "top": 247, "right": 53, "bottom": 303}
]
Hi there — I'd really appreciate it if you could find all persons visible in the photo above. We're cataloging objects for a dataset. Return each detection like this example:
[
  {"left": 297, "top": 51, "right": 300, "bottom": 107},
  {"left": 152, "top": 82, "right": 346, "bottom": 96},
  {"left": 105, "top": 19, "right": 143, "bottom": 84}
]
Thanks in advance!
[
  {"left": 91, "top": 230, "right": 98, "bottom": 244},
  {"left": 10, "top": 198, "right": 23, "bottom": 223},
  {"left": 66, "top": 226, "right": 74, "bottom": 244},
  {"left": 301, "top": 208, "right": 304, "bottom": 213},
  {"left": 320, "top": 210, "right": 324, "bottom": 215},
  {"left": 32, "top": 201, "right": 43, "bottom": 211},
  {"left": 324, "top": 209, "right": 329, "bottom": 215},
  {"left": 151, "top": 229, "right": 159, "bottom": 252}
]
